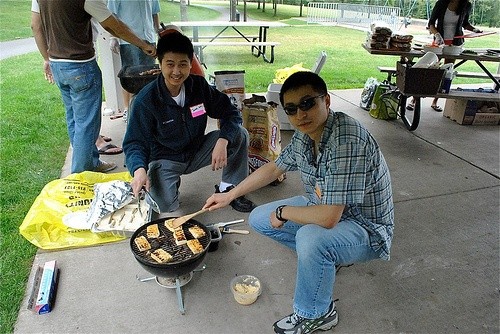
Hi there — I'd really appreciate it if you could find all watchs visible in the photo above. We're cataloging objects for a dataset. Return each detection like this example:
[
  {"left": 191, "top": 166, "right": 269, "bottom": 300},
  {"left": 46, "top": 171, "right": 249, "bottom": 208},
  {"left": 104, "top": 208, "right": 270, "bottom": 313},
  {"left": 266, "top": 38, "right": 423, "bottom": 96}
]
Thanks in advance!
[{"left": 276, "top": 205, "right": 288, "bottom": 223}]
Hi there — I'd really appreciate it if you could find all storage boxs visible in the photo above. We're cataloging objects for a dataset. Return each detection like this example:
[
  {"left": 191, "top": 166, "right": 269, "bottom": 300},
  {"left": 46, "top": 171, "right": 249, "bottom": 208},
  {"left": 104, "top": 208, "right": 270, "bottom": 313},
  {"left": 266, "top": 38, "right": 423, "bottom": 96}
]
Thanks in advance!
[
  {"left": 396, "top": 61, "right": 446, "bottom": 94},
  {"left": 267, "top": 83, "right": 295, "bottom": 130}
]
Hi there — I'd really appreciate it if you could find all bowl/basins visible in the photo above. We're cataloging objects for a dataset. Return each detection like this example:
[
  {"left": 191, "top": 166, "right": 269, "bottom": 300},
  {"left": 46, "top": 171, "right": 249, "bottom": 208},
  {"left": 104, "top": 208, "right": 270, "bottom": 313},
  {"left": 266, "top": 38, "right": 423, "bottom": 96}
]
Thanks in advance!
[
  {"left": 443, "top": 45, "right": 463, "bottom": 56},
  {"left": 230, "top": 275, "right": 262, "bottom": 305},
  {"left": 424, "top": 44, "right": 442, "bottom": 54}
]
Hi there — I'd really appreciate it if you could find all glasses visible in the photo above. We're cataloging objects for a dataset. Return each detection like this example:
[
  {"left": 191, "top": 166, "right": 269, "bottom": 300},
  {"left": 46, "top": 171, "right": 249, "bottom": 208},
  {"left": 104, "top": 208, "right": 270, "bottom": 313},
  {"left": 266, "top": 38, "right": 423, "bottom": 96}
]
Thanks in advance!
[{"left": 283, "top": 93, "right": 325, "bottom": 115}]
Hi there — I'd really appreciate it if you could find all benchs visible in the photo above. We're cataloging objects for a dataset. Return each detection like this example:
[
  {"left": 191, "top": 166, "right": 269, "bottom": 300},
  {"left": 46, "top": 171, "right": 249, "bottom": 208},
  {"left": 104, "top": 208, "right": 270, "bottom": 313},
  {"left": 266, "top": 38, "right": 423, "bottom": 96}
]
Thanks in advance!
[
  {"left": 188, "top": 35, "right": 281, "bottom": 69},
  {"left": 377, "top": 66, "right": 500, "bottom": 131}
]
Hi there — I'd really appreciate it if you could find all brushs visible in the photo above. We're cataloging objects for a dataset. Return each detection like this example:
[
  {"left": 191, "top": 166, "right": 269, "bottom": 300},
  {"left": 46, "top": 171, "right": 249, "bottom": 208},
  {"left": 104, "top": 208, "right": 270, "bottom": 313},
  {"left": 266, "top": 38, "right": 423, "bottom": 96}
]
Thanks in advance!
[
  {"left": 219, "top": 228, "right": 249, "bottom": 235},
  {"left": 214, "top": 220, "right": 245, "bottom": 227}
]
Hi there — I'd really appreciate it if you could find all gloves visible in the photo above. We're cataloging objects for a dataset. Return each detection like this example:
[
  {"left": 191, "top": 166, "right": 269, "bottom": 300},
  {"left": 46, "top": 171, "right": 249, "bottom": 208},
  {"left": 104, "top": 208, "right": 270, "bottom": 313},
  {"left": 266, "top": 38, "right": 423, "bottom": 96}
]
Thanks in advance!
[{"left": 109, "top": 37, "right": 120, "bottom": 54}]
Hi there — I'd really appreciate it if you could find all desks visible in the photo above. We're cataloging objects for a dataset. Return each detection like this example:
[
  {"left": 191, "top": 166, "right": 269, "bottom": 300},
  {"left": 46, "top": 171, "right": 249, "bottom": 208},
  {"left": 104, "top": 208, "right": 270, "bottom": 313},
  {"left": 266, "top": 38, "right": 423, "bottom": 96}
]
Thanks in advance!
[
  {"left": 171, "top": 22, "right": 290, "bottom": 55},
  {"left": 362, "top": 44, "right": 500, "bottom": 88}
]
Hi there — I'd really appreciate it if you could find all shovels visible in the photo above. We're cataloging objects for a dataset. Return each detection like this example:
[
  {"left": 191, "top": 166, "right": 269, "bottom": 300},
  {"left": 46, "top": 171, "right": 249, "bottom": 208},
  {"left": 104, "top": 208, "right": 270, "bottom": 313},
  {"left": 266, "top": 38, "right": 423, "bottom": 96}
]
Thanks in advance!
[{"left": 172, "top": 207, "right": 210, "bottom": 229}]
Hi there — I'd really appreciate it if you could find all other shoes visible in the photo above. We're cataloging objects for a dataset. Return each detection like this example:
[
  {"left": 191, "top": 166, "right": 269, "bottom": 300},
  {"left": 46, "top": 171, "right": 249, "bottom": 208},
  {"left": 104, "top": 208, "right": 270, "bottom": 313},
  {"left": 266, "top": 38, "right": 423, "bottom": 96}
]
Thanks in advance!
[
  {"left": 407, "top": 99, "right": 414, "bottom": 110},
  {"left": 431, "top": 104, "right": 443, "bottom": 112},
  {"left": 96, "top": 161, "right": 116, "bottom": 172},
  {"left": 214, "top": 184, "right": 257, "bottom": 212}
]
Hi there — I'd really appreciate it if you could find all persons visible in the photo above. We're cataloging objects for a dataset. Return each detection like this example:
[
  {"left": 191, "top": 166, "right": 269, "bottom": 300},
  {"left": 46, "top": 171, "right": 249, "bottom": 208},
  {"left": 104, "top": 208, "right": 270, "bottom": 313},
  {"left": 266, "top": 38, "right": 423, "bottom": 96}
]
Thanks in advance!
[
  {"left": 122, "top": 33, "right": 256, "bottom": 212},
  {"left": 201, "top": 71, "right": 395, "bottom": 334},
  {"left": 405, "top": 16, "right": 411, "bottom": 27},
  {"left": 32, "top": 0, "right": 161, "bottom": 172},
  {"left": 406, "top": 0, "right": 483, "bottom": 112}
]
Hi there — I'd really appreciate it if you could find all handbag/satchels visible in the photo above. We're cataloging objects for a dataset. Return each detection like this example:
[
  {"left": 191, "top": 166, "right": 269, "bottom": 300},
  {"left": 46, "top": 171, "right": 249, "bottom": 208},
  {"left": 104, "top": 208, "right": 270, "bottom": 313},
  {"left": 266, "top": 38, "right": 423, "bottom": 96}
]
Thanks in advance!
[{"left": 359, "top": 76, "right": 402, "bottom": 120}]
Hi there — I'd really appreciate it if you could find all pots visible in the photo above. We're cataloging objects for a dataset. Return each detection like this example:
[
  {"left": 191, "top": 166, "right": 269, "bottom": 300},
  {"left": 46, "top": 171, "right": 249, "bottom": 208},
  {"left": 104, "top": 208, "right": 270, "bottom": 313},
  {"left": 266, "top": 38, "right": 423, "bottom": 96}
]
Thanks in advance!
[
  {"left": 118, "top": 64, "right": 161, "bottom": 94},
  {"left": 130, "top": 216, "right": 212, "bottom": 278}
]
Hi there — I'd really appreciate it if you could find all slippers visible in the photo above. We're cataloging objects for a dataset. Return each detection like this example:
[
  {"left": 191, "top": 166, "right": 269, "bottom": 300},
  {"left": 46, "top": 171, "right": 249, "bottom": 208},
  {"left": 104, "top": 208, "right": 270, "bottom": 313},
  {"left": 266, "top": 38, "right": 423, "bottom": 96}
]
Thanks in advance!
[{"left": 97, "top": 134, "right": 122, "bottom": 155}]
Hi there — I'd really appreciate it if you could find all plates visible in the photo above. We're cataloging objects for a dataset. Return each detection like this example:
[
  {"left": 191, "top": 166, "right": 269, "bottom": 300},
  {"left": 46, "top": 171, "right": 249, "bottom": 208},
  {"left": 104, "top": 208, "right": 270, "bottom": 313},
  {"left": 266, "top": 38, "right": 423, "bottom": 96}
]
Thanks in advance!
[{"left": 413, "top": 45, "right": 500, "bottom": 58}]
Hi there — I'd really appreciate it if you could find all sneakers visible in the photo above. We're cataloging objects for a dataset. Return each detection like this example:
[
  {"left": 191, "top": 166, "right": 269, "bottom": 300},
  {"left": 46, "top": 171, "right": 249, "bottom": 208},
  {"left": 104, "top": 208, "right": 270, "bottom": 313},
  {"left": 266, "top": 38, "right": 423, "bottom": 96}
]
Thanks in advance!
[{"left": 272, "top": 298, "right": 339, "bottom": 334}]
